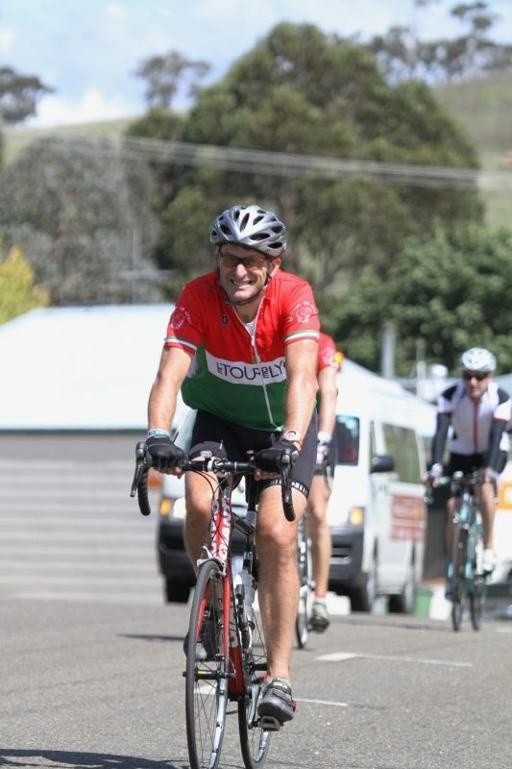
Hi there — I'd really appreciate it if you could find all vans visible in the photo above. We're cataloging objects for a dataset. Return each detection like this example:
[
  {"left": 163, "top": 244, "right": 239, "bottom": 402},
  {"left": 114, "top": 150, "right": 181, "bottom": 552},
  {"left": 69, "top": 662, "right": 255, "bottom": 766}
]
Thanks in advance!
[{"left": 157, "top": 391, "right": 431, "bottom": 615}]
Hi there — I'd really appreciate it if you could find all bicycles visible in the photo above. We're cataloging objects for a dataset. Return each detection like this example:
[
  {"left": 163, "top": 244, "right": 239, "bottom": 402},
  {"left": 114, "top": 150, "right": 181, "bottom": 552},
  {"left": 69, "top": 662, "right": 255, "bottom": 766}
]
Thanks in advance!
[
  {"left": 130, "top": 428, "right": 296, "bottom": 764},
  {"left": 229, "top": 452, "right": 330, "bottom": 650},
  {"left": 424, "top": 467, "right": 494, "bottom": 635}
]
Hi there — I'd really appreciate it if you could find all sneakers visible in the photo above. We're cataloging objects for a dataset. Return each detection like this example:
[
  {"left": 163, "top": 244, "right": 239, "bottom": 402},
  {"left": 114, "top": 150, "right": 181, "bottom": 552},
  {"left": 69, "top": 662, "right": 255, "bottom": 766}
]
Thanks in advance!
[
  {"left": 482, "top": 548, "right": 498, "bottom": 573},
  {"left": 309, "top": 601, "right": 329, "bottom": 632},
  {"left": 183, "top": 608, "right": 220, "bottom": 661},
  {"left": 259, "top": 679, "right": 295, "bottom": 720}
]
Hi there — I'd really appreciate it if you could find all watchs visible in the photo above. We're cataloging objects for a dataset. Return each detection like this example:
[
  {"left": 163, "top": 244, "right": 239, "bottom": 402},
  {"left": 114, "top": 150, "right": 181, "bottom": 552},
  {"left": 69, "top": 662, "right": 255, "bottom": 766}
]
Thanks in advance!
[{"left": 279, "top": 430, "right": 304, "bottom": 449}]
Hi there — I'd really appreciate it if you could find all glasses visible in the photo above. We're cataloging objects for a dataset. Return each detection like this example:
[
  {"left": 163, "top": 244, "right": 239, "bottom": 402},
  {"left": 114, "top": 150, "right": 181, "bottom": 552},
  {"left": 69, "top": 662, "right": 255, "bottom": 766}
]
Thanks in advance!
[
  {"left": 219, "top": 245, "right": 270, "bottom": 268},
  {"left": 463, "top": 369, "right": 490, "bottom": 380}
]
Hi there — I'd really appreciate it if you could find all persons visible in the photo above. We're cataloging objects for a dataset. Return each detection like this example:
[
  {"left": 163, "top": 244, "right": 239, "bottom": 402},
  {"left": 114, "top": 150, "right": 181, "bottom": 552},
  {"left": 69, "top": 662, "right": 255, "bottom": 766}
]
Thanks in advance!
[
  {"left": 148, "top": 205, "right": 320, "bottom": 722},
  {"left": 306, "top": 332, "right": 337, "bottom": 630},
  {"left": 430, "top": 347, "right": 512, "bottom": 602}
]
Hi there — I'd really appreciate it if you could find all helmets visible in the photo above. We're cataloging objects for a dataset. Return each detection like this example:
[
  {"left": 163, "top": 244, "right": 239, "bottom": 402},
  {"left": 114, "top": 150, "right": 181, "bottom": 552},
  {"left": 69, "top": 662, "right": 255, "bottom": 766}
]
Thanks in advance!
[
  {"left": 209, "top": 204, "right": 288, "bottom": 258},
  {"left": 460, "top": 347, "right": 496, "bottom": 372}
]
